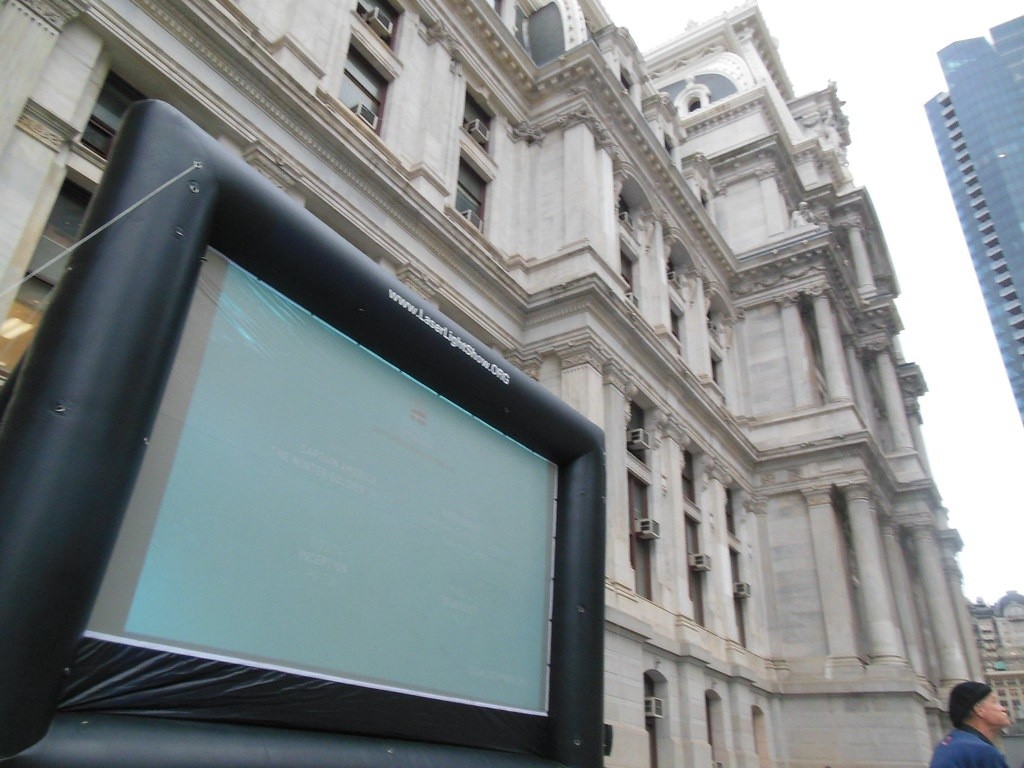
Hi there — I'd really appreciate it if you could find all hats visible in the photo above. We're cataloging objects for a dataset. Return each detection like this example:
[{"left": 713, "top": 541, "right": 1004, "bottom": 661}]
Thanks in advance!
[{"left": 950, "top": 682, "right": 991, "bottom": 727}]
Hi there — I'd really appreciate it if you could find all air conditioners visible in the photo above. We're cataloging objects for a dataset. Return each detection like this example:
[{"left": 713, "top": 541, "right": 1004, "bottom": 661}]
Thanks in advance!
[
  {"left": 619, "top": 212, "right": 632, "bottom": 229},
  {"left": 627, "top": 293, "right": 638, "bottom": 308},
  {"left": 627, "top": 428, "right": 651, "bottom": 450},
  {"left": 644, "top": 697, "right": 664, "bottom": 719},
  {"left": 689, "top": 554, "right": 711, "bottom": 571},
  {"left": 733, "top": 583, "right": 751, "bottom": 599},
  {"left": 366, "top": 8, "right": 393, "bottom": 37},
  {"left": 347, "top": 102, "right": 378, "bottom": 130},
  {"left": 464, "top": 119, "right": 490, "bottom": 144},
  {"left": 707, "top": 321, "right": 717, "bottom": 335},
  {"left": 668, "top": 272, "right": 681, "bottom": 289},
  {"left": 635, "top": 518, "right": 660, "bottom": 540},
  {"left": 461, "top": 209, "right": 483, "bottom": 233}
]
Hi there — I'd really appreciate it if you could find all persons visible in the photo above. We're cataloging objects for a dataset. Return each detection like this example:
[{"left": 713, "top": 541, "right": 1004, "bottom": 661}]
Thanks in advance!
[{"left": 928, "top": 680, "right": 1013, "bottom": 768}]
